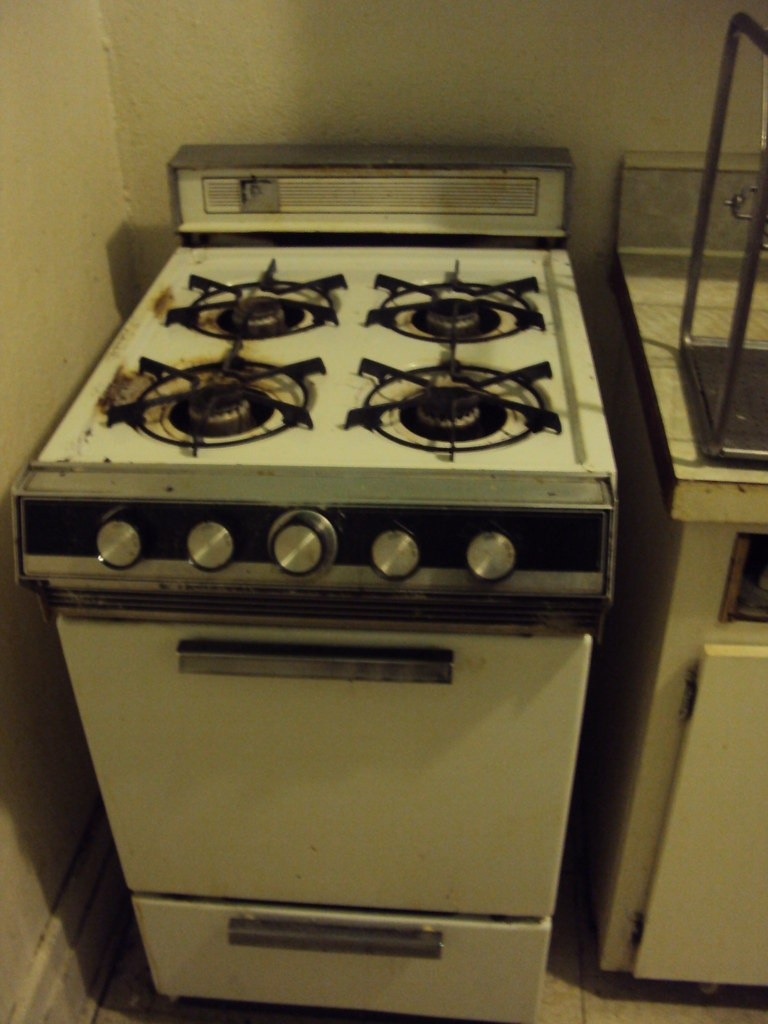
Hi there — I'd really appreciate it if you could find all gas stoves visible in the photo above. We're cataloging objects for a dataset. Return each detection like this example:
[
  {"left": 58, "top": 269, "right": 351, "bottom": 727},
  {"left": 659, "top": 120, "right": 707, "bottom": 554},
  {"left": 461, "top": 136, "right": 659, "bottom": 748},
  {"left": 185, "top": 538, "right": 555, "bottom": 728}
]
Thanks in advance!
[{"left": 10, "top": 145, "right": 619, "bottom": 596}]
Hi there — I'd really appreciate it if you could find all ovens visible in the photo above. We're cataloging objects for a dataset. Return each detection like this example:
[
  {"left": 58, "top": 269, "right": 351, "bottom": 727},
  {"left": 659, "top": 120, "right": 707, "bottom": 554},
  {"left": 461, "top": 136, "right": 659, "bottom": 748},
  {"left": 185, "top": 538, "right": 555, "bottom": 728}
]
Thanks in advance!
[{"left": 52, "top": 603, "right": 601, "bottom": 1024}]
